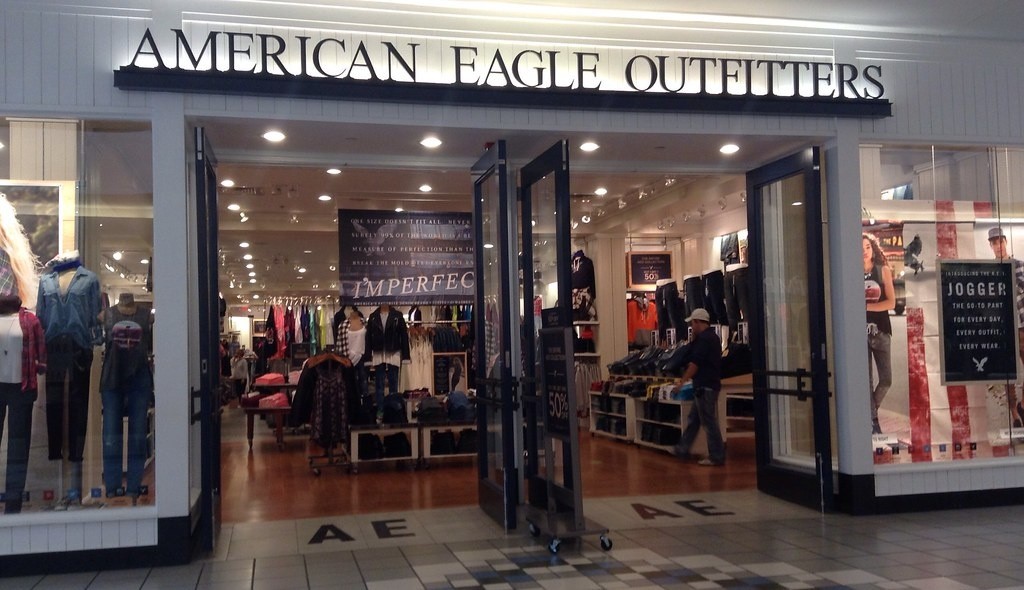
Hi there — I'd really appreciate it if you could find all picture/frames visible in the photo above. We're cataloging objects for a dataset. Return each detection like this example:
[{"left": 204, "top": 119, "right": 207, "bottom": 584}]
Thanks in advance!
[{"left": 432, "top": 352, "right": 468, "bottom": 397}]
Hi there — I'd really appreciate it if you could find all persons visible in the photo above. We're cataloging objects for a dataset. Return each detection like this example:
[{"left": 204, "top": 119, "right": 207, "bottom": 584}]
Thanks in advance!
[
  {"left": 0, "top": 294, "right": 49, "bottom": 513},
  {"left": 218, "top": 328, "right": 277, "bottom": 380},
  {"left": 725, "top": 263, "right": 751, "bottom": 344},
  {"left": 333, "top": 298, "right": 364, "bottom": 344},
  {"left": 862, "top": 232, "right": 896, "bottom": 435},
  {"left": 684, "top": 274, "right": 705, "bottom": 319},
  {"left": 100, "top": 292, "right": 154, "bottom": 504},
  {"left": 336, "top": 311, "right": 372, "bottom": 417},
  {"left": 655, "top": 279, "right": 687, "bottom": 344},
  {"left": 230, "top": 349, "right": 248, "bottom": 408},
  {"left": 365, "top": 304, "right": 412, "bottom": 405},
  {"left": 668, "top": 308, "right": 725, "bottom": 466},
  {"left": 702, "top": 268, "right": 729, "bottom": 357},
  {"left": 36, "top": 249, "right": 106, "bottom": 512},
  {"left": 989, "top": 227, "right": 1024, "bottom": 427}
]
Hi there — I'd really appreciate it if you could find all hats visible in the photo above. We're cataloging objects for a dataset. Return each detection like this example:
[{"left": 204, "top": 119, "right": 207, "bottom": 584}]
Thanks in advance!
[
  {"left": 684, "top": 309, "right": 709, "bottom": 322},
  {"left": 988, "top": 228, "right": 1005, "bottom": 239}
]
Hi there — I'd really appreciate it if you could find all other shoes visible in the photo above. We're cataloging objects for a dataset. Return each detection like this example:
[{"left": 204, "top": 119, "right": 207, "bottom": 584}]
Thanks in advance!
[
  {"left": 422, "top": 388, "right": 431, "bottom": 398},
  {"left": 699, "top": 459, "right": 714, "bottom": 465},
  {"left": 665, "top": 447, "right": 676, "bottom": 455},
  {"left": 414, "top": 389, "right": 421, "bottom": 398},
  {"left": 1013, "top": 419, "right": 1021, "bottom": 428},
  {"left": 1018, "top": 403, "right": 1024, "bottom": 426},
  {"left": 403, "top": 390, "right": 413, "bottom": 400}
]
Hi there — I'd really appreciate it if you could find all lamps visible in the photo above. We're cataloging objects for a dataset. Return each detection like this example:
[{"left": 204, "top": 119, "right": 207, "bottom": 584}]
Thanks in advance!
[
  {"left": 667, "top": 215, "right": 678, "bottom": 228},
  {"left": 581, "top": 215, "right": 592, "bottom": 225},
  {"left": 664, "top": 176, "right": 678, "bottom": 188},
  {"left": 290, "top": 214, "right": 299, "bottom": 224},
  {"left": 218, "top": 249, "right": 250, "bottom": 304},
  {"left": 697, "top": 204, "right": 706, "bottom": 217},
  {"left": 718, "top": 197, "right": 727, "bottom": 211},
  {"left": 740, "top": 192, "right": 747, "bottom": 202},
  {"left": 657, "top": 220, "right": 666, "bottom": 231},
  {"left": 597, "top": 207, "right": 608, "bottom": 217},
  {"left": 572, "top": 222, "right": 579, "bottom": 230},
  {"left": 617, "top": 199, "right": 628, "bottom": 210},
  {"left": 638, "top": 185, "right": 656, "bottom": 200},
  {"left": 240, "top": 212, "right": 250, "bottom": 223},
  {"left": 100, "top": 256, "right": 147, "bottom": 283},
  {"left": 682, "top": 210, "right": 691, "bottom": 223}
]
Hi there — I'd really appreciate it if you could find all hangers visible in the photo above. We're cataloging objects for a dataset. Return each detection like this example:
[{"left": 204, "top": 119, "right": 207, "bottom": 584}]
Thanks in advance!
[
  {"left": 307, "top": 345, "right": 351, "bottom": 368},
  {"left": 484, "top": 294, "right": 497, "bottom": 303},
  {"left": 406, "top": 320, "right": 457, "bottom": 337},
  {"left": 268, "top": 293, "right": 337, "bottom": 306}
]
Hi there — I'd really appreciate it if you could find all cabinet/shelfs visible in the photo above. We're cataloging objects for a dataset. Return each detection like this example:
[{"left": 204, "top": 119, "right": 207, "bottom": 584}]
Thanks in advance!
[
  {"left": 244, "top": 382, "right": 299, "bottom": 452},
  {"left": 631, "top": 397, "right": 707, "bottom": 455},
  {"left": 587, "top": 390, "right": 634, "bottom": 444},
  {"left": 419, "top": 418, "right": 556, "bottom": 470},
  {"left": 347, "top": 423, "right": 420, "bottom": 475}
]
